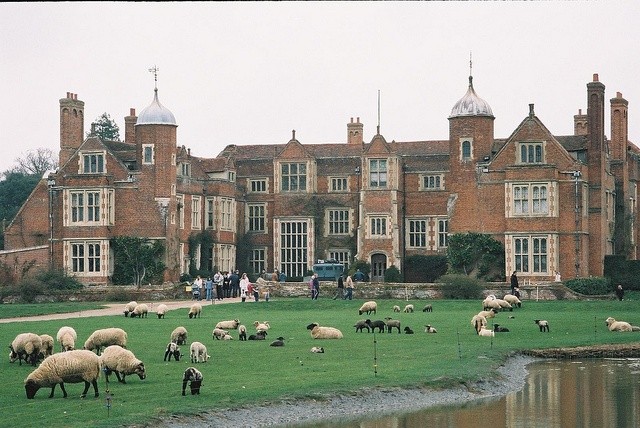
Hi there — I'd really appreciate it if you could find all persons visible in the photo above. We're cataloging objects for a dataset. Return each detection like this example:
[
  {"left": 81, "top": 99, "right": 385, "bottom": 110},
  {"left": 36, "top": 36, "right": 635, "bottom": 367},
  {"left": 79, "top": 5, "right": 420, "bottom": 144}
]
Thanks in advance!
[
  {"left": 333, "top": 273, "right": 346, "bottom": 300},
  {"left": 510, "top": 270, "right": 519, "bottom": 305},
  {"left": 343, "top": 272, "right": 353, "bottom": 300},
  {"left": 195, "top": 269, "right": 250, "bottom": 303},
  {"left": 310, "top": 276, "right": 319, "bottom": 300},
  {"left": 313, "top": 273, "right": 319, "bottom": 301},
  {"left": 352, "top": 268, "right": 365, "bottom": 282},
  {"left": 260, "top": 269, "right": 287, "bottom": 281}
]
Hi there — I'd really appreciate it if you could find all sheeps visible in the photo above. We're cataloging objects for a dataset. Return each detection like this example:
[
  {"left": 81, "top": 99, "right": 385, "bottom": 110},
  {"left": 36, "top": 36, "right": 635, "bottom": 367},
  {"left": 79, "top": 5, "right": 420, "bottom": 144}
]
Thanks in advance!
[
  {"left": 365, "top": 318, "right": 386, "bottom": 332},
  {"left": 393, "top": 304, "right": 400, "bottom": 311},
  {"left": 214, "top": 318, "right": 241, "bottom": 329},
  {"left": 56, "top": 326, "right": 77, "bottom": 352},
  {"left": 182, "top": 366, "right": 203, "bottom": 395},
  {"left": 404, "top": 326, "right": 414, "bottom": 334},
  {"left": 359, "top": 300, "right": 377, "bottom": 314},
  {"left": 472, "top": 314, "right": 488, "bottom": 333},
  {"left": 384, "top": 316, "right": 401, "bottom": 333},
  {"left": 156, "top": 303, "right": 169, "bottom": 318},
  {"left": 248, "top": 330, "right": 268, "bottom": 340},
  {"left": 253, "top": 320, "right": 268, "bottom": 329},
  {"left": 188, "top": 303, "right": 202, "bottom": 318},
  {"left": 263, "top": 320, "right": 271, "bottom": 329},
  {"left": 163, "top": 342, "right": 183, "bottom": 360},
  {"left": 212, "top": 327, "right": 230, "bottom": 339},
  {"left": 479, "top": 325, "right": 495, "bottom": 337},
  {"left": 482, "top": 299, "right": 502, "bottom": 312},
  {"left": 239, "top": 324, "right": 247, "bottom": 340},
  {"left": 123, "top": 300, "right": 138, "bottom": 316},
  {"left": 220, "top": 331, "right": 233, "bottom": 339},
  {"left": 424, "top": 324, "right": 437, "bottom": 332},
  {"left": 493, "top": 298, "right": 513, "bottom": 311},
  {"left": 270, "top": 335, "right": 285, "bottom": 346},
  {"left": 100, "top": 344, "right": 147, "bottom": 384},
  {"left": 10, "top": 332, "right": 42, "bottom": 366},
  {"left": 404, "top": 304, "right": 414, "bottom": 312},
  {"left": 83, "top": 327, "right": 128, "bottom": 355},
  {"left": 534, "top": 319, "right": 549, "bottom": 332},
  {"left": 23, "top": 349, "right": 102, "bottom": 399},
  {"left": 477, "top": 307, "right": 498, "bottom": 318},
  {"left": 130, "top": 303, "right": 148, "bottom": 318},
  {"left": 171, "top": 326, "right": 188, "bottom": 344},
  {"left": 306, "top": 322, "right": 344, "bottom": 338},
  {"left": 353, "top": 319, "right": 370, "bottom": 332},
  {"left": 493, "top": 323, "right": 510, "bottom": 332},
  {"left": 423, "top": 303, "right": 432, "bottom": 312},
  {"left": 39, "top": 333, "right": 54, "bottom": 358},
  {"left": 190, "top": 340, "right": 210, "bottom": 362},
  {"left": 504, "top": 294, "right": 522, "bottom": 308},
  {"left": 605, "top": 317, "right": 633, "bottom": 331}
]
